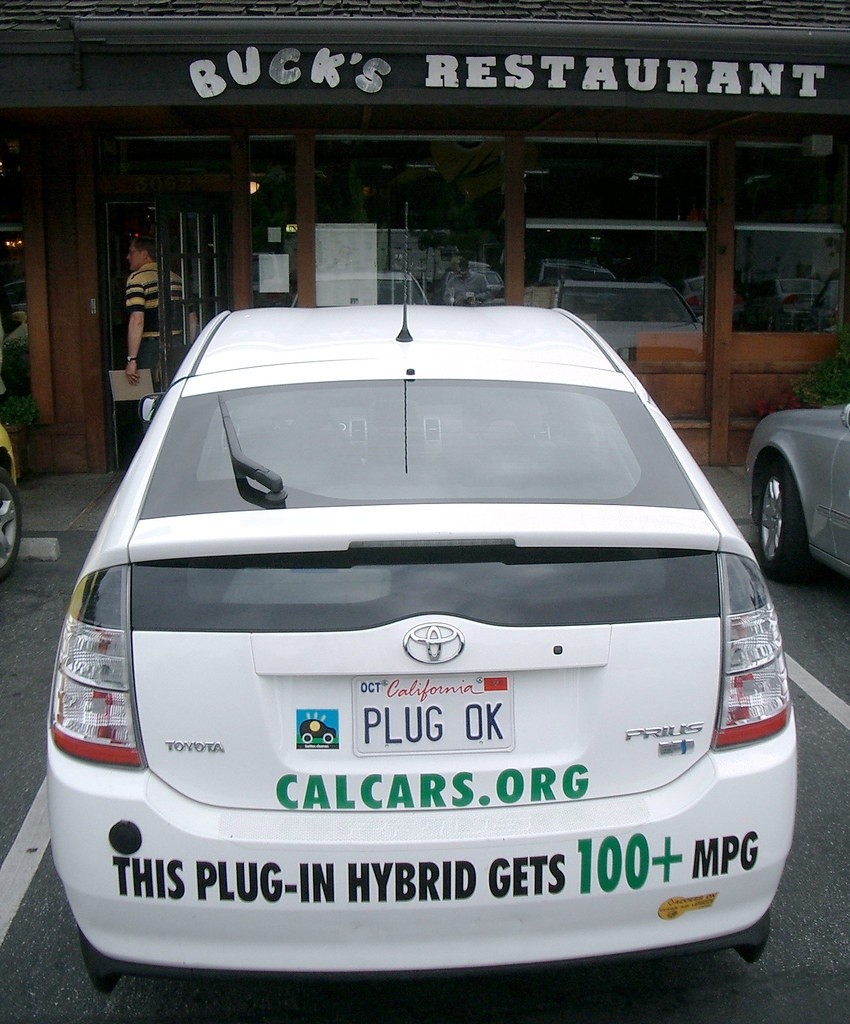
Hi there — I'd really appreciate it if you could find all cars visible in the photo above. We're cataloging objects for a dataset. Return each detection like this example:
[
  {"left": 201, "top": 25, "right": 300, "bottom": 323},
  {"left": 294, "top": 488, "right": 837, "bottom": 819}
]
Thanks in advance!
[
  {"left": 374, "top": 263, "right": 842, "bottom": 335},
  {"left": 743, "top": 400, "right": 850, "bottom": 588},
  {"left": 0, "top": 424, "right": 25, "bottom": 585},
  {"left": 48, "top": 304, "right": 796, "bottom": 996}
]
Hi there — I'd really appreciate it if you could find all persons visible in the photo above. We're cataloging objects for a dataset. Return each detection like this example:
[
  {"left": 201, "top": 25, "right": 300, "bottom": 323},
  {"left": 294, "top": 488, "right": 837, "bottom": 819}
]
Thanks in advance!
[
  {"left": 443, "top": 254, "right": 491, "bottom": 306},
  {"left": 124, "top": 233, "right": 196, "bottom": 434}
]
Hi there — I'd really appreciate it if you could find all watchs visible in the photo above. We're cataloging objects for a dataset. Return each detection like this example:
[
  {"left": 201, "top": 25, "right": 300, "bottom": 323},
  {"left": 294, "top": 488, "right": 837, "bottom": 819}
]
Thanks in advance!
[{"left": 126, "top": 356, "right": 137, "bottom": 362}]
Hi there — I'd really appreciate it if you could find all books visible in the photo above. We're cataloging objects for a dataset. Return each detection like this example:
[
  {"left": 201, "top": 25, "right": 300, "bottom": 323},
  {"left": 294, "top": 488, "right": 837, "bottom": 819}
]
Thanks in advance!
[{"left": 109, "top": 369, "right": 153, "bottom": 401}]
